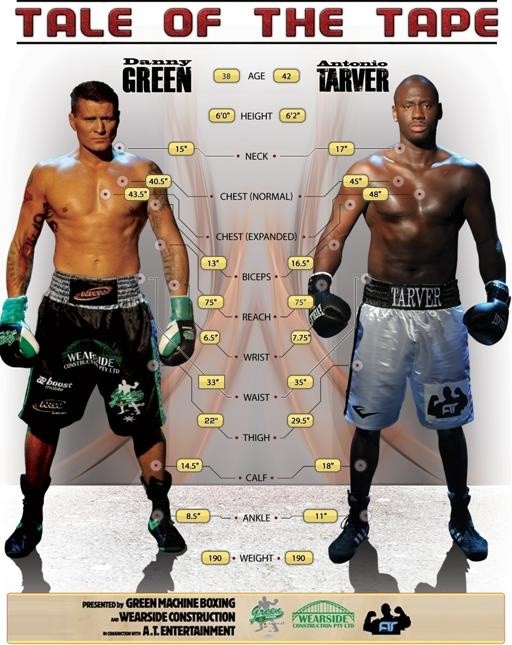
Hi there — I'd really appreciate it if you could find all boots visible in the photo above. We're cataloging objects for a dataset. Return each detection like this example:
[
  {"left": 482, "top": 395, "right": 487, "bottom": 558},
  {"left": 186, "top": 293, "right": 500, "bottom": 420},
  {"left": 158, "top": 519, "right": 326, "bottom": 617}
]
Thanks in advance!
[
  {"left": 450, "top": 493, "right": 488, "bottom": 562},
  {"left": 330, "top": 496, "right": 369, "bottom": 562}
]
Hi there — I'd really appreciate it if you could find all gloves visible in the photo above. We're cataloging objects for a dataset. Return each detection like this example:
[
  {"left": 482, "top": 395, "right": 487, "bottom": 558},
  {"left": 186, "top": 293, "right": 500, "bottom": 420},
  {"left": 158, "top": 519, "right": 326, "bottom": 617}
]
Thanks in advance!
[
  {"left": 160, "top": 297, "right": 196, "bottom": 366},
  {"left": 462, "top": 280, "right": 509, "bottom": 346},
  {"left": 308, "top": 273, "right": 350, "bottom": 337},
  {"left": 0, "top": 295, "right": 41, "bottom": 367}
]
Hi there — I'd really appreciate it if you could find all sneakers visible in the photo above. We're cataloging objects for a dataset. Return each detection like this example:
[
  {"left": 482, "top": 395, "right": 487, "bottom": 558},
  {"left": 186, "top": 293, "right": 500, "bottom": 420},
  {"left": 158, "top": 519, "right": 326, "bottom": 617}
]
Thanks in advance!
[
  {"left": 5, "top": 509, "right": 42, "bottom": 557},
  {"left": 147, "top": 512, "right": 187, "bottom": 552}
]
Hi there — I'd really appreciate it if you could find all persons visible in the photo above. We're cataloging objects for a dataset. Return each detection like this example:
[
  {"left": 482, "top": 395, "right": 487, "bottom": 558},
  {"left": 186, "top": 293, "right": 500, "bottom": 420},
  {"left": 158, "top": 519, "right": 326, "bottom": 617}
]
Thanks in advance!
[
  {"left": 308, "top": 75, "right": 509, "bottom": 565},
  {"left": 1, "top": 80, "right": 197, "bottom": 558}
]
